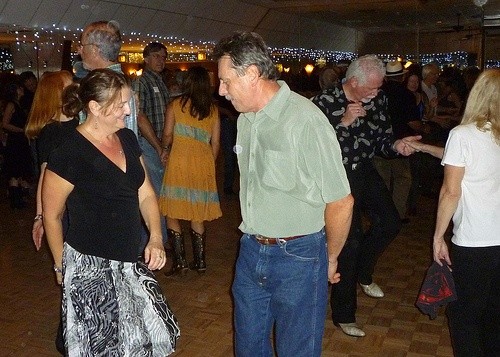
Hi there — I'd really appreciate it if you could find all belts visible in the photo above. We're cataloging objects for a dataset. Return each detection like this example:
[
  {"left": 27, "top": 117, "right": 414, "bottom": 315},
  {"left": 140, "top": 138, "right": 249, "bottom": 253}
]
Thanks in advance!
[{"left": 253, "top": 233, "right": 306, "bottom": 244}]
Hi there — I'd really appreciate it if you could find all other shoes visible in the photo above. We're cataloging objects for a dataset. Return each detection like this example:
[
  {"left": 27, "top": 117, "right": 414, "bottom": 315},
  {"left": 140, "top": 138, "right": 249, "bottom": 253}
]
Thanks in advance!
[
  {"left": 359, "top": 282, "right": 385, "bottom": 298},
  {"left": 337, "top": 322, "right": 367, "bottom": 337}
]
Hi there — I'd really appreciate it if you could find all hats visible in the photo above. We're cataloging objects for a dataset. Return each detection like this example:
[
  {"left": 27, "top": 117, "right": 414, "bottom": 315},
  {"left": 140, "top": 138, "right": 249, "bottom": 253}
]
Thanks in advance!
[{"left": 386, "top": 61, "right": 410, "bottom": 76}]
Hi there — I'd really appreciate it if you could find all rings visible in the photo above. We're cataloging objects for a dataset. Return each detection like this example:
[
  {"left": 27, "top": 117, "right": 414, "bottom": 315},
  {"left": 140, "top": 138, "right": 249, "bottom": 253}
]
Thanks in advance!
[{"left": 356, "top": 109, "right": 359, "bottom": 113}]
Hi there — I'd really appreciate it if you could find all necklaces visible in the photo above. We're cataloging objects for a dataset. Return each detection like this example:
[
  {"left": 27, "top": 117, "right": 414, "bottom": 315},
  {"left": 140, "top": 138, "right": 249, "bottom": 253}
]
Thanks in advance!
[{"left": 83, "top": 123, "right": 124, "bottom": 157}]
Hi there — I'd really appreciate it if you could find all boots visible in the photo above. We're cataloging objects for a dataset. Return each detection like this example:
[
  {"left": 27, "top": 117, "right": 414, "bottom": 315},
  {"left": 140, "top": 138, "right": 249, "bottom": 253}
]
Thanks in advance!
[
  {"left": 9, "top": 185, "right": 26, "bottom": 209},
  {"left": 189, "top": 226, "right": 207, "bottom": 273},
  {"left": 164, "top": 228, "right": 189, "bottom": 276}
]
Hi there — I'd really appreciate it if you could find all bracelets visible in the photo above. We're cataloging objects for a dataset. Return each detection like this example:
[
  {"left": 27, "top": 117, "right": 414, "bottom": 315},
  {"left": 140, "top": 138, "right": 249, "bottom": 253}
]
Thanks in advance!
[
  {"left": 34, "top": 215, "right": 43, "bottom": 221},
  {"left": 54, "top": 264, "right": 62, "bottom": 273}
]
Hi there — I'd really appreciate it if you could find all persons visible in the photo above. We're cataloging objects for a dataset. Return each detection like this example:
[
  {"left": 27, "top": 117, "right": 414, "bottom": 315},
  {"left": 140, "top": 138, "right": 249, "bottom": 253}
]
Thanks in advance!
[
  {"left": 25, "top": 72, "right": 80, "bottom": 354},
  {"left": 42, "top": 68, "right": 180, "bottom": 357},
  {"left": 80, "top": 21, "right": 138, "bottom": 139},
  {"left": 215, "top": 31, "right": 355, "bottom": 357},
  {"left": 433, "top": 69, "right": 500, "bottom": 357},
  {"left": 0, "top": 70, "right": 38, "bottom": 207},
  {"left": 373, "top": 61, "right": 461, "bottom": 222},
  {"left": 130, "top": 42, "right": 239, "bottom": 276},
  {"left": 275, "top": 66, "right": 344, "bottom": 89},
  {"left": 315, "top": 55, "right": 422, "bottom": 336}
]
